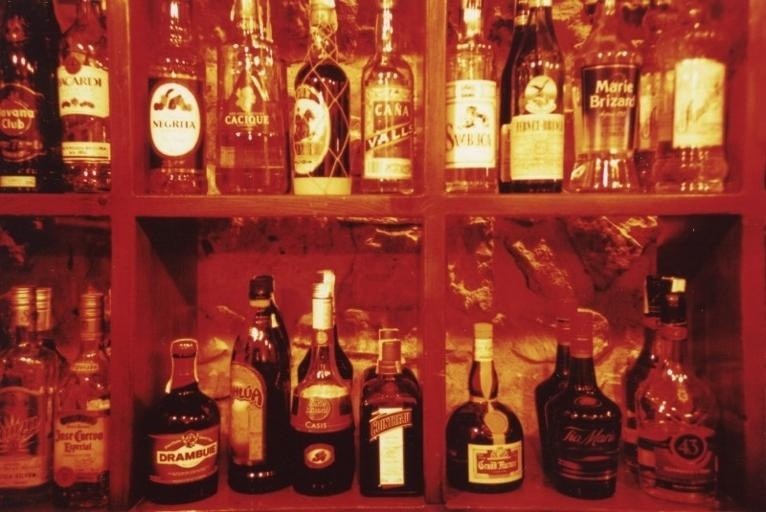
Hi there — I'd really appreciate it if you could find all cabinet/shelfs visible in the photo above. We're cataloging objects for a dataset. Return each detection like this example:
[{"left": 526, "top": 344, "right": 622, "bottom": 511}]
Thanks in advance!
[{"left": 0, "top": 0, "right": 766, "bottom": 511}]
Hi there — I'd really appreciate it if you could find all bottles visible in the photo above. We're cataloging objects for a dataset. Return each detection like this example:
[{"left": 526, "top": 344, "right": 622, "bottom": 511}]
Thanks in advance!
[
  {"left": 0, "top": 267, "right": 720, "bottom": 512},
  {"left": 0, "top": 0, "right": 732, "bottom": 195}
]
